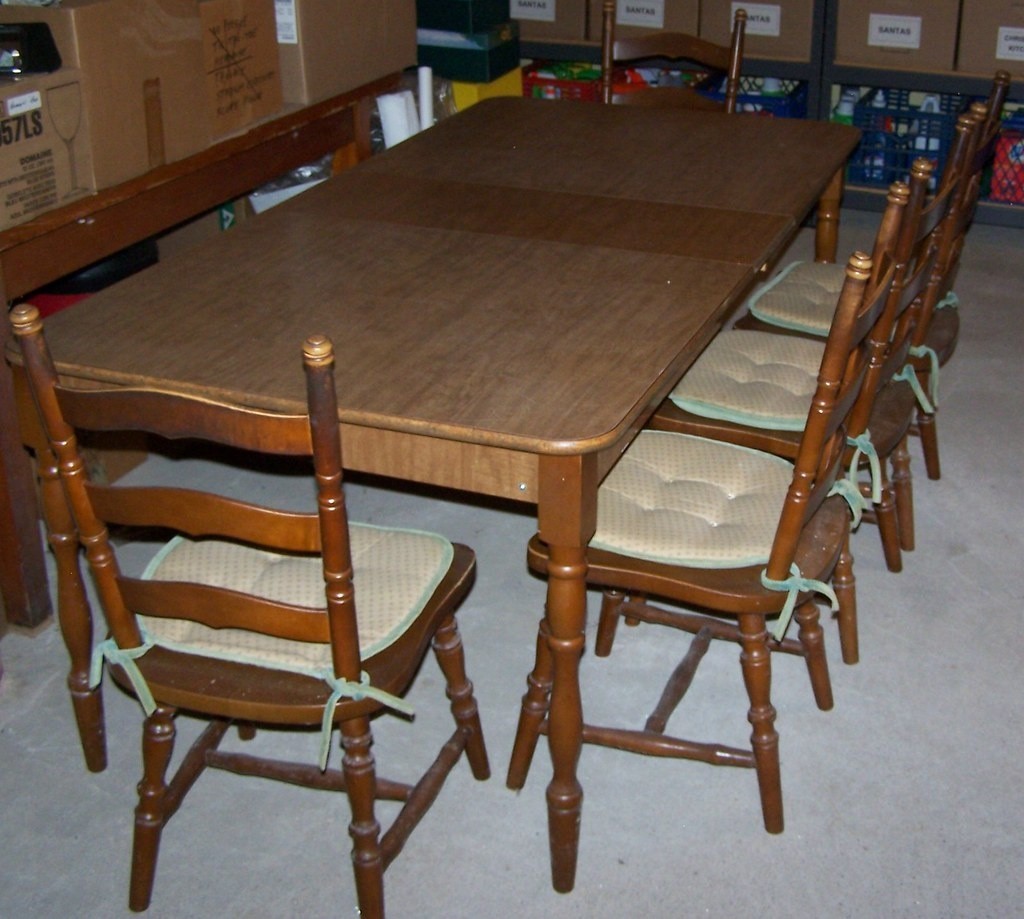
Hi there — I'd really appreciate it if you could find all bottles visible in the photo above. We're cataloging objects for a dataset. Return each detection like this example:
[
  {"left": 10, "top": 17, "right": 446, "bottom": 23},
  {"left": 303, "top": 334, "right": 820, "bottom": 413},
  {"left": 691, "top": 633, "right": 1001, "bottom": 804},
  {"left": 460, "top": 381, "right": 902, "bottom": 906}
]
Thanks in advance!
[
  {"left": 864, "top": 89, "right": 890, "bottom": 180},
  {"left": 905, "top": 93, "right": 940, "bottom": 187},
  {"left": 743, "top": 78, "right": 784, "bottom": 115},
  {"left": 831, "top": 86, "right": 860, "bottom": 182}
]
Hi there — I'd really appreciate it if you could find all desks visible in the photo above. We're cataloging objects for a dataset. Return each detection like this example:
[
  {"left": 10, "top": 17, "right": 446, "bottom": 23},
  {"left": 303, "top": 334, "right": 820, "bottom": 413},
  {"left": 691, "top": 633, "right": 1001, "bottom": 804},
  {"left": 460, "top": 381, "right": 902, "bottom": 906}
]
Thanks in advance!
[
  {"left": 0, "top": 94, "right": 836, "bottom": 893},
  {"left": 0, "top": 67, "right": 418, "bottom": 641}
]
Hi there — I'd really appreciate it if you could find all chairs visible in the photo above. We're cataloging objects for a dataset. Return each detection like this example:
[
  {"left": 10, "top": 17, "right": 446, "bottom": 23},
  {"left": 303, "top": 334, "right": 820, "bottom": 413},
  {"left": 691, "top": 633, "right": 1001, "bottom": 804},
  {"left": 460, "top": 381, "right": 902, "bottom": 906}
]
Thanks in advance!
[
  {"left": 9, "top": 304, "right": 491, "bottom": 919},
  {"left": 503, "top": 71, "right": 1009, "bottom": 833}
]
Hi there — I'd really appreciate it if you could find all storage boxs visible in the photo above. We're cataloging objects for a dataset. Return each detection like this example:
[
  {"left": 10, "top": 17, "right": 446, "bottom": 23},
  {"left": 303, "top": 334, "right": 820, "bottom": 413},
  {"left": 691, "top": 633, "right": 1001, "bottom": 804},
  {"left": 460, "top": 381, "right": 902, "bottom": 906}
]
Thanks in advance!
[
  {"left": 0, "top": 0, "right": 283, "bottom": 192},
  {"left": 275, "top": 0, "right": 417, "bottom": 106},
  {"left": 509, "top": 0, "right": 1024, "bottom": 205},
  {"left": 0, "top": 66, "right": 96, "bottom": 231}
]
